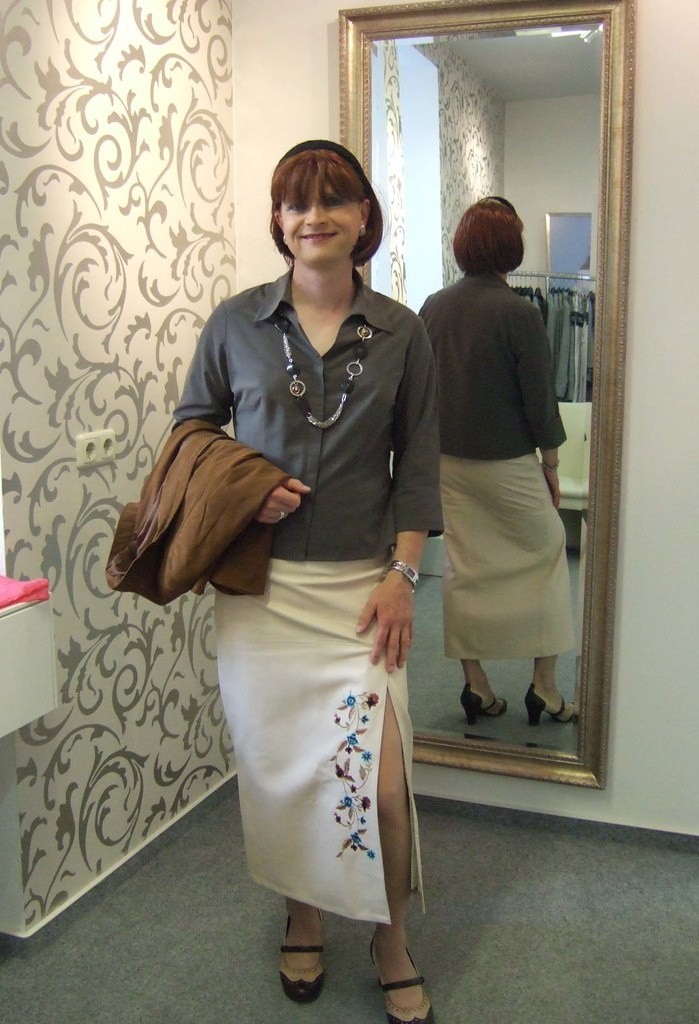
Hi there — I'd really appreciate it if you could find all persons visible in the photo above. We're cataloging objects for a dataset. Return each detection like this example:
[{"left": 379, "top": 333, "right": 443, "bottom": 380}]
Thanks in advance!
[
  {"left": 170, "top": 138, "right": 443, "bottom": 1021},
  {"left": 418, "top": 196, "right": 577, "bottom": 728}
]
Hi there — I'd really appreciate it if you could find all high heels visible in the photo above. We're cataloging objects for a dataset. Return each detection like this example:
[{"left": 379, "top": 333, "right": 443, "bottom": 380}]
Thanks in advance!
[
  {"left": 525, "top": 685, "right": 574, "bottom": 726},
  {"left": 370, "top": 933, "right": 435, "bottom": 1023},
  {"left": 460, "top": 682, "right": 507, "bottom": 725},
  {"left": 279, "top": 905, "right": 323, "bottom": 1004}
]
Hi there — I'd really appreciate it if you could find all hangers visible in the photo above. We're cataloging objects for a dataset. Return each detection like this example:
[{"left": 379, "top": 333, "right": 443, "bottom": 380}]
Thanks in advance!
[{"left": 506, "top": 271, "right": 596, "bottom": 299}]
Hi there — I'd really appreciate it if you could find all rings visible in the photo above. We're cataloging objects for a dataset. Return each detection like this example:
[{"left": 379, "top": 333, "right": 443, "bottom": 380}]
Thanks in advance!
[{"left": 277, "top": 510, "right": 285, "bottom": 520}]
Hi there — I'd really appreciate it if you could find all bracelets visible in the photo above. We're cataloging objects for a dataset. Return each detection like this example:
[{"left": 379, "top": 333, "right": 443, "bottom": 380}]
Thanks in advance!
[
  {"left": 387, "top": 558, "right": 420, "bottom": 591},
  {"left": 540, "top": 458, "right": 560, "bottom": 472}
]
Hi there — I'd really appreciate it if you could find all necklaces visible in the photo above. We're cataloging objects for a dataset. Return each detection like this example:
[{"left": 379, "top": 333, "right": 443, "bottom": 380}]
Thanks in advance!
[{"left": 271, "top": 306, "right": 374, "bottom": 431}]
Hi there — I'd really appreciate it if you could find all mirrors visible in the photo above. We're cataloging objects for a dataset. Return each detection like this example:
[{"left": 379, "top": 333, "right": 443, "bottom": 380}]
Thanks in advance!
[{"left": 338, "top": 0, "right": 635, "bottom": 790}]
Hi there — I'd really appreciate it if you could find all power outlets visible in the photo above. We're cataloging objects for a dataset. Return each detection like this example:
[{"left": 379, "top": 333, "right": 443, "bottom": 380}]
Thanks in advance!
[{"left": 76, "top": 429, "right": 116, "bottom": 472}]
